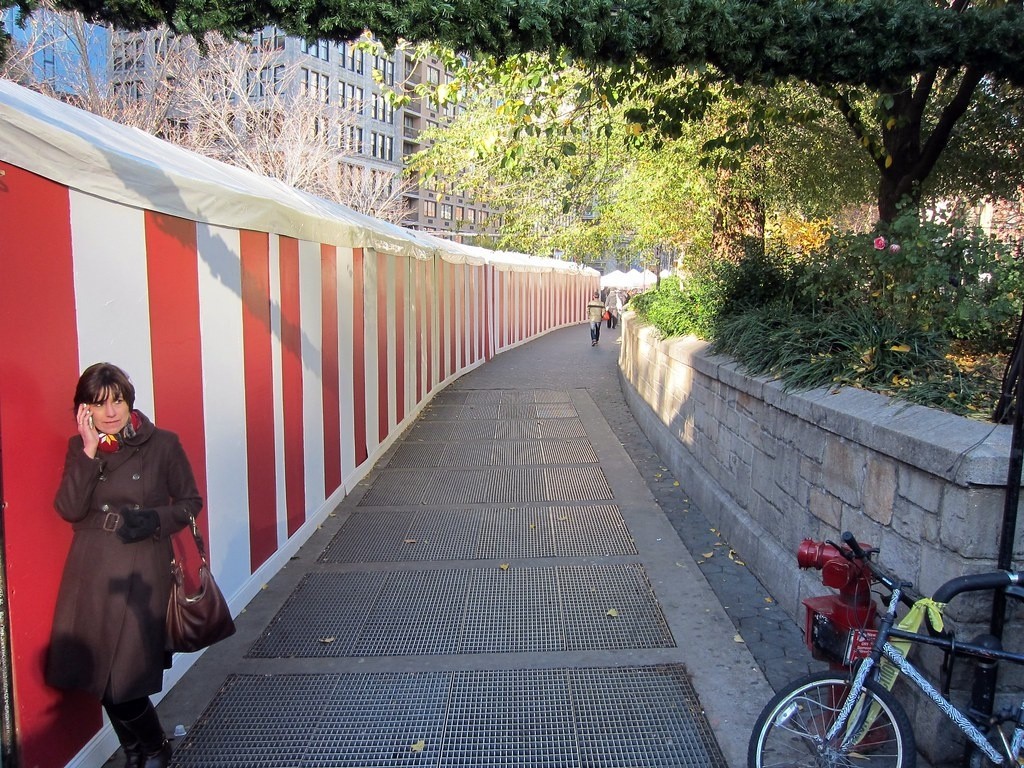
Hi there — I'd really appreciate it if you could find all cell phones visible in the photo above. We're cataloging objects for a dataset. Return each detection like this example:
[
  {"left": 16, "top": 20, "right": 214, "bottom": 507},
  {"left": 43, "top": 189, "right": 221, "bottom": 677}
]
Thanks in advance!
[{"left": 82, "top": 403, "right": 95, "bottom": 429}]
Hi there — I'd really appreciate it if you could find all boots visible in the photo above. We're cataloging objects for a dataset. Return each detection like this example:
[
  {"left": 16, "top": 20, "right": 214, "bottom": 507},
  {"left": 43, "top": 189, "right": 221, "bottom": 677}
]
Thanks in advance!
[
  {"left": 120, "top": 699, "right": 174, "bottom": 768},
  {"left": 111, "top": 719, "right": 145, "bottom": 768}
]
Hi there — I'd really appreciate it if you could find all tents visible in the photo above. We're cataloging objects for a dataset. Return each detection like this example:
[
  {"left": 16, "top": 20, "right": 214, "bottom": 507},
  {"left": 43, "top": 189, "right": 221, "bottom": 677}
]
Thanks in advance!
[{"left": 599, "top": 268, "right": 672, "bottom": 290}]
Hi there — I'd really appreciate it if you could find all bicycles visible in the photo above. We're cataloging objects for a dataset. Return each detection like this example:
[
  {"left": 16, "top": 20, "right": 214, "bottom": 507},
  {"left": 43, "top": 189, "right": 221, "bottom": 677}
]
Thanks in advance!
[{"left": 746, "top": 531, "right": 1024, "bottom": 768}]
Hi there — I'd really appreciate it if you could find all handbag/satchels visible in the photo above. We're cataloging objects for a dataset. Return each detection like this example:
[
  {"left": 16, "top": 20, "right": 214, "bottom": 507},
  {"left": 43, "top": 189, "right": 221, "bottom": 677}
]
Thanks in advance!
[
  {"left": 165, "top": 507, "right": 237, "bottom": 652},
  {"left": 602, "top": 311, "right": 610, "bottom": 320}
]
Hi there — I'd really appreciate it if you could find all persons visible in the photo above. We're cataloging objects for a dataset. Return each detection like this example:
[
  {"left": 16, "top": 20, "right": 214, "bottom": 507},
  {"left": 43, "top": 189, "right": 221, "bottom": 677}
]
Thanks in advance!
[
  {"left": 587, "top": 292, "right": 606, "bottom": 347},
  {"left": 42, "top": 362, "right": 204, "bottom": 768},
  {"left": 601, "top": 286, "right": 648, "bottom": 330}
]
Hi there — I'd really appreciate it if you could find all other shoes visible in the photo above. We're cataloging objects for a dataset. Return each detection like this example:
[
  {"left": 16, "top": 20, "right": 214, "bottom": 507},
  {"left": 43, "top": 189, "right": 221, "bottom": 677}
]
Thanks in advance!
[
  {"left": 597, "top": 341, "right": 599, "bottom": 344},
  {"left": 592, "top": 341, "right": 596, "bottom": 347},
  {"left": 612, "top": 326, "right": 616, "bottom": 329}
]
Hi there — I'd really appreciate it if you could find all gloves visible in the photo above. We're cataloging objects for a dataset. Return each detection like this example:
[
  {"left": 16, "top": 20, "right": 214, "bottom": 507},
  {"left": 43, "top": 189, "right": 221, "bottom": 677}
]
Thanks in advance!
[{"left": 116, "top": 508, "right": 159, "bottom": 541}]
